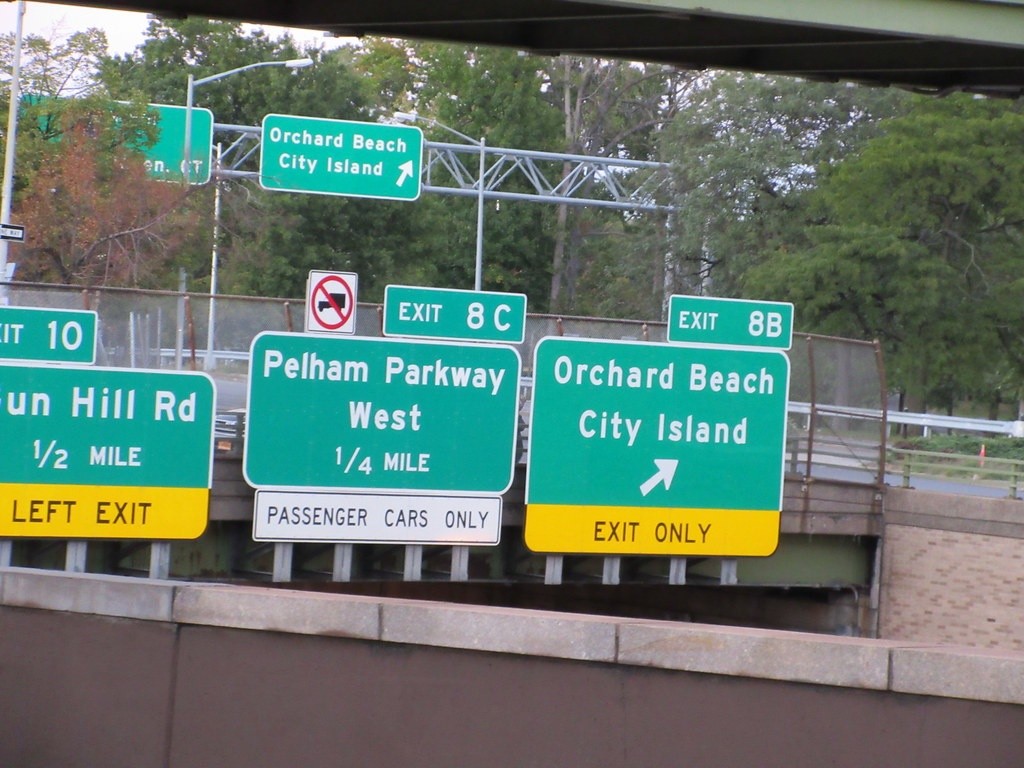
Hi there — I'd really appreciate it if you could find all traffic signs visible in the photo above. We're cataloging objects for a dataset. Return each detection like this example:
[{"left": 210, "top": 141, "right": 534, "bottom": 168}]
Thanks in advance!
[
  {"left": 241, "top": 343, "right": 528, "bottom": 495},
  {"left": 523, "top": 344, "right": 796, "bottom": 559},
  {"left": 261, "top": 111, "right": 425, "bottom": 202},
  {"left": 0, "top": 361, "right": 220, "bottom": 541}
]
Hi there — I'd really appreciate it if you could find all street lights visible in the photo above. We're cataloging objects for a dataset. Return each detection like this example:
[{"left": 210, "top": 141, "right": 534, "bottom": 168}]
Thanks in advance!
[
  {"left": 393, "top": 112, "right": 485, "bottom": 291},
  {"left": 178, "top": 59, "right": 313, "bottom": 191}
]
